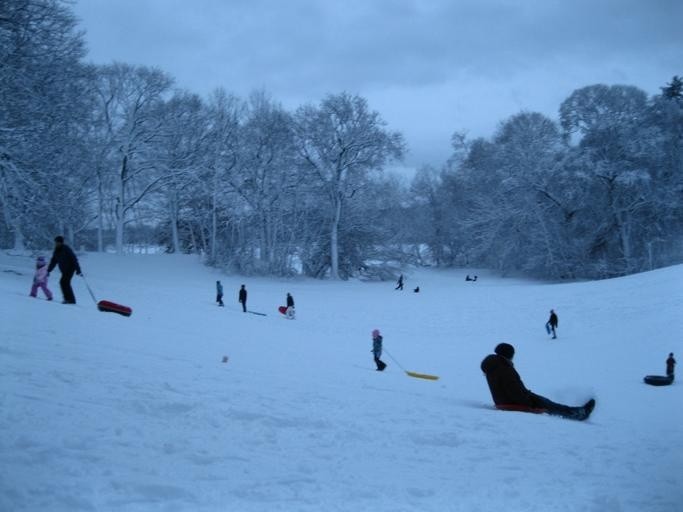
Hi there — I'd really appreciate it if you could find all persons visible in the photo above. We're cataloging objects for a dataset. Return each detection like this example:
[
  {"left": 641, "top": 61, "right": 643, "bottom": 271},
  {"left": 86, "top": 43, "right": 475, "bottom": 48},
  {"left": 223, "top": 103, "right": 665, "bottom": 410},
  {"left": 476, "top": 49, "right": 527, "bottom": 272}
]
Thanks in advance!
[
  {"left": 47, "top": 235, "right": 84, "bottom": 305},
  {"left": 480, "top": 343, "right": 595, "bottom": 421},
  {"left": 395, "top": 274, "right": 404, "bottom": 290},
  {"left": 239, "top": 285, "right": 247, "bottom": 312},
  {"left": 546, "top": 309, "right": 558, "bottom": 339},
  {"left": 371, "top": 330, "right": 387, "bottom": 372},
  {"left": 413, "top": 286, "right": 419, "bottom": 292},
  {"left": 666, "top": 352, "right": 676, "bottom": 376},
  {"left": 29, "top": 255, "right": 53, "bottom": 301},
  {"left": 216, "top": 281, "right": 224, "bottom": 306},
  {"left": 286, "top": 292, "right": 295, "bottom": 320}
]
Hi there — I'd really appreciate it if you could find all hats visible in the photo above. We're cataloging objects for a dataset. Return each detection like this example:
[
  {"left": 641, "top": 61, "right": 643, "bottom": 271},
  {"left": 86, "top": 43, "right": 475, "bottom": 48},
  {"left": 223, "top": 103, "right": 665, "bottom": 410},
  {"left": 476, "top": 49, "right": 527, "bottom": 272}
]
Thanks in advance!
[
  {"left": 55, "top": 236, "right": 63, "bottom": 243},
  {"left": 36, "top": 255, "right": 45, "bottom": 266},
  {"left": 372, "top": 329, "right": 380, "bottom": 336},
  {"left": 494, "top": 342, "right": 516, "bottom": 360}
]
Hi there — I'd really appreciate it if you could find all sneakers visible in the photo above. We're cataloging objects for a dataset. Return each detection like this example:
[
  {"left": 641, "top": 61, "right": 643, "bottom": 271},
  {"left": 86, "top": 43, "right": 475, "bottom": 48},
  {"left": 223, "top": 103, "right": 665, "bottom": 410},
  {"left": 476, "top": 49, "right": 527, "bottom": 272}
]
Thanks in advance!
[{"left": 576, "top": 398, "right": 595, "bottom": 421}]
